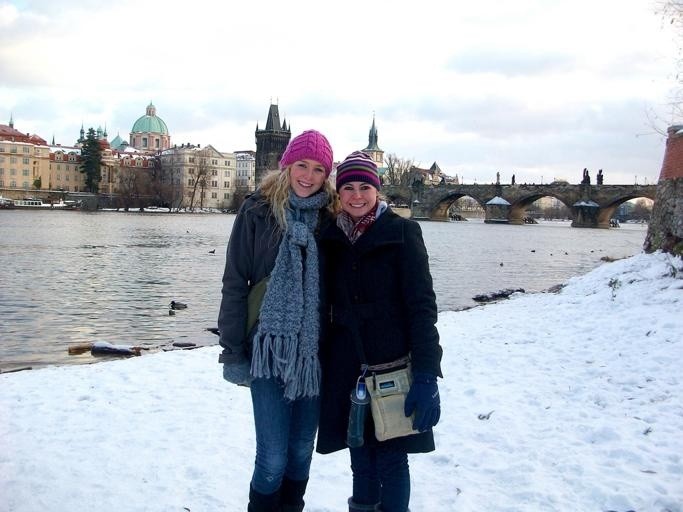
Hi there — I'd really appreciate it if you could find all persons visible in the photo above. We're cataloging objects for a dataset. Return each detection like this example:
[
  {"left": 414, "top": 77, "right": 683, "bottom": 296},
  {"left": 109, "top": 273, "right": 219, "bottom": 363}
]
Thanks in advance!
[
  {"left": 315, "top": 150, "right": 443, "bottom": 512},
  {"left": 218, "top": 129, "right": 343, "bottom": 512}
]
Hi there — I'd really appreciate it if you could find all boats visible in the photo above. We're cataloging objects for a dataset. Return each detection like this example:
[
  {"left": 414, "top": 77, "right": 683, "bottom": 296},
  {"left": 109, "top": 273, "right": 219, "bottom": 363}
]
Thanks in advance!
[{"left": 0, "top": 197, "right": 79, "bottom": 211}]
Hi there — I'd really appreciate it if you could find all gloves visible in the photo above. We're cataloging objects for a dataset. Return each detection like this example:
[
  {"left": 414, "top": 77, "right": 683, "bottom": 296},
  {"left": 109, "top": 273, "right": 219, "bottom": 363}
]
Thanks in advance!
[
  {"left": 223, "top": 362, "right": 256, "bottom": 383},
  {"left": 405, "top": 372, "right": 440, "bottom": 433}
]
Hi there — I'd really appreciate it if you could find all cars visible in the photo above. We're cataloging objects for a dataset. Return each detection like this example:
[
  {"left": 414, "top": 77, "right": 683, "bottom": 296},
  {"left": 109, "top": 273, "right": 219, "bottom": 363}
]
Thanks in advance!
[{"left": 388, "top": 200, "right": 408, "bottom": 208}]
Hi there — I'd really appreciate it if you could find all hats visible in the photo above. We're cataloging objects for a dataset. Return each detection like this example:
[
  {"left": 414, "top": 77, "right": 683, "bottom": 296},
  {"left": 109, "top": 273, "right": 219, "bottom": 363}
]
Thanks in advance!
[
  {"left": 280, "top": 129, "right": 333, "bottom": 180},
  {"left": 336, "top": 150, "right": 381, "bottom": 194}
]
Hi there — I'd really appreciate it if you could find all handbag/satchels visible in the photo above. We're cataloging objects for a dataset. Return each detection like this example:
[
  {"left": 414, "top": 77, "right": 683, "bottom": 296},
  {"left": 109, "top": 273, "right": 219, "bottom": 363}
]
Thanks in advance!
[{"left": 365, "top": 364, "right": 428, "bottom": 442}]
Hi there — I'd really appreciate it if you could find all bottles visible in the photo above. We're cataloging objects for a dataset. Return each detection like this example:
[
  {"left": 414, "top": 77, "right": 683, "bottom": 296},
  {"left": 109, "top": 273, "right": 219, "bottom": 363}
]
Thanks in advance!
[{"left": 347, "top": 386, "right": 367, "bottom": 448}]
